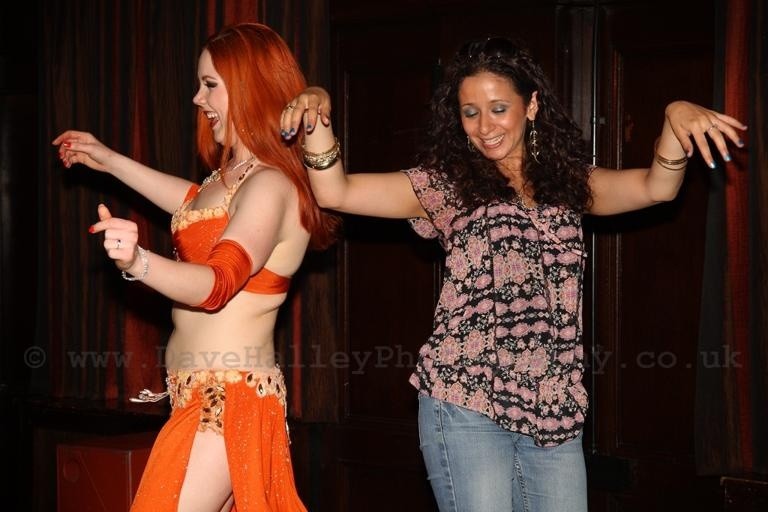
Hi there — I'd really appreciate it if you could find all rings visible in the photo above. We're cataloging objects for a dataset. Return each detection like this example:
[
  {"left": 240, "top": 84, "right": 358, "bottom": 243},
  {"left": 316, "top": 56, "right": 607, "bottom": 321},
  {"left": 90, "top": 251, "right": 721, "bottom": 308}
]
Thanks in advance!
[
  {"left": 282, "top": 104, "right": 295, "bottom": 113},
  {"left": 706, "top": 125, "right": 717, "bottom": 135},
  {"left": 114, "top": 239, "right": 123, "bottom": 249}
]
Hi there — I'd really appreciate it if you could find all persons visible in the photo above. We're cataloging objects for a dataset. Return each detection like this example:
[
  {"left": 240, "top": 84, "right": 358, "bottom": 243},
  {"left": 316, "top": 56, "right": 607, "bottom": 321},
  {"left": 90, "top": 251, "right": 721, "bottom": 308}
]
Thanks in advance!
[
  {"left": 53, "top": 19, "right": 344, "bottom": 510},
  {"left": 278, "top": 36, "right": 755, "bottom": 510}
]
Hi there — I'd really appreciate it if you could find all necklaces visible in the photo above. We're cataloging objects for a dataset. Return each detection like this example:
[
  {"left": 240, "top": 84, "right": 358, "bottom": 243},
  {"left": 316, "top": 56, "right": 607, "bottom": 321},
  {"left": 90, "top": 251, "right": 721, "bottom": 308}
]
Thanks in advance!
[{"left": 197, "top": 155, "right": 252, "bottom": 192}]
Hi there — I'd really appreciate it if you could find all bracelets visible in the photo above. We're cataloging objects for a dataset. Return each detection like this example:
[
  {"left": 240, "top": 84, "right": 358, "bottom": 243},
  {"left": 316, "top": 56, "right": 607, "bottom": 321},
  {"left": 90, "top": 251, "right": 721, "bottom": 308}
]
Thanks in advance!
[
  {"left": 121, "top": 245, "right": 150, "bottom": 280},
  {"left": 654, "top": 136, "right": 689, "bottom": 172},
  {"left": 300, "top": 138, "right": 342, "bottom": 171}
]
